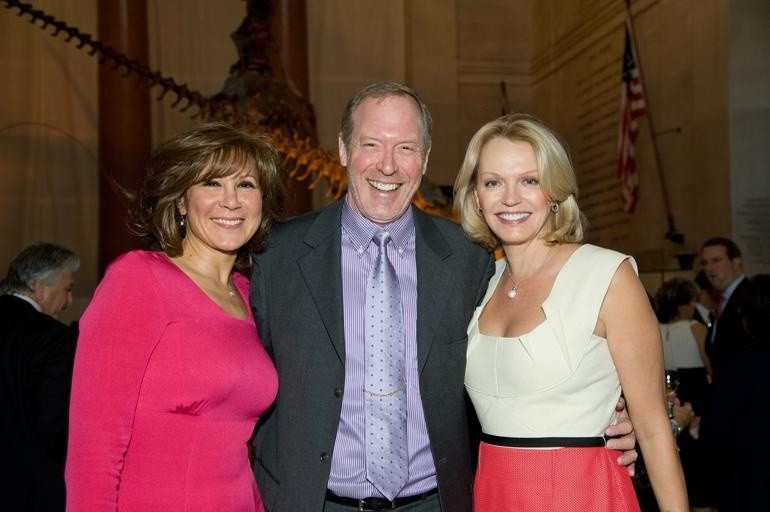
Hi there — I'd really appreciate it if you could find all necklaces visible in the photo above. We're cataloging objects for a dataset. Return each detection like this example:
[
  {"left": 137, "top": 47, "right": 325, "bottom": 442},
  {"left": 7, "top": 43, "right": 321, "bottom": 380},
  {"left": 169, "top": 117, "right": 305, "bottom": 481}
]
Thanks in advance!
[
  {"left": 180, "top": 254, "right": 238, "bottom": 298},
  {"left": 505, "top": 241, "right": 554, "bottom": 300}
]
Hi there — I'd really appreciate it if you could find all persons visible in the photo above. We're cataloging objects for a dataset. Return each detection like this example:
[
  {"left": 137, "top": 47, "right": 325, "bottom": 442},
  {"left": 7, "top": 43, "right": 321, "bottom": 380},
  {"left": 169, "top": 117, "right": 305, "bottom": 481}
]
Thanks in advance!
[
  {"left": 452, "top": 116, "right": 689, "bottom": 512},
  {"left": 250, "top": 81, "right": 637, "bottom": 512},
  {"left": 0, "top": 242, "right": 86, "bottom": 512},
  {"left": 62, "top": 122, "right": 280, "bottom": 511},
  {"left": 649, "top": 236, "right": 770, "bottom": 512}
]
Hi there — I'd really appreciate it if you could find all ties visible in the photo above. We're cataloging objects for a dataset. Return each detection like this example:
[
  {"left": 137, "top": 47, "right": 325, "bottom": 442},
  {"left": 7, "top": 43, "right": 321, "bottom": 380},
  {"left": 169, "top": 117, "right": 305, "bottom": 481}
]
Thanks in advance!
[{"left": 365, "top": 231, "right": 408, "bottom": 501}]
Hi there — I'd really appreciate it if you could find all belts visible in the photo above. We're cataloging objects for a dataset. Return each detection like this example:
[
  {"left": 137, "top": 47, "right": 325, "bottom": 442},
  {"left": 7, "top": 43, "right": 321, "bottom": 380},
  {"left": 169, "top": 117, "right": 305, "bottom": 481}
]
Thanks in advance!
[{"left": 326, "top": 488, "right": 438, "bottom": 510}]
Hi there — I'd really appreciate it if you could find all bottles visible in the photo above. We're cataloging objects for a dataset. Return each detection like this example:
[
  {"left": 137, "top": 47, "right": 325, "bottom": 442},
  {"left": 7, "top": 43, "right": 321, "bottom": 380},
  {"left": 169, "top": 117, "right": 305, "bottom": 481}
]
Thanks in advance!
[{"left": 660, "top": 370, "right": 680, "bottom": 449}]
